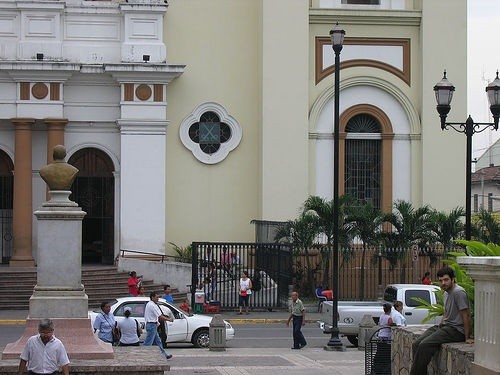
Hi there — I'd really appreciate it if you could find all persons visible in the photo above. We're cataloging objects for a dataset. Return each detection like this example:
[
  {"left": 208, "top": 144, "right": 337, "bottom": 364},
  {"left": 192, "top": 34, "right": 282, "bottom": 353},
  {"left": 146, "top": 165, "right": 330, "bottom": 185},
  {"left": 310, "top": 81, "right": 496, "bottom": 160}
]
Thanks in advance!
[
  {"left": 118, "top": 307, "right": 143, "bottom": 346},
  {"left": 93, "top": 302, "right": 115, "bottom": 346},
  {"left": 137, "top": 286, "right": 145, "bottom": 297},
  {"left": 422, "top": 272, "right": 431, "bottom": 285},
  {"left": 143, "top": 291, "right": 172, "bottom": 359},
  {"left": 390, "top": 301, "right": 407, "bottom": 326},
  {"left": 162, "top": 285, "right": 173, "bottom": 304},
  {"left": 236, "top": 271, "right": 252, "bottom": 315},
  {"left": 128, "top": 271, "right": 139, "bottom": 297},
  {"left": 18, "top": 319, "right": 71, "bottom": 375},
  {"left": 410, "top": 267, "right": 474, "bottom": 375},
  {"left": 371, "top": 303, "right": 395, "bottom": 375},
  {"left": 286, "top": 292, "right": 307, "bottom": 349},
  {"left": 39, "top": 145, "right": 79, "bottom": 191},
  {"left": 204, "top": 247, "right": 241, "bottom": 270}
]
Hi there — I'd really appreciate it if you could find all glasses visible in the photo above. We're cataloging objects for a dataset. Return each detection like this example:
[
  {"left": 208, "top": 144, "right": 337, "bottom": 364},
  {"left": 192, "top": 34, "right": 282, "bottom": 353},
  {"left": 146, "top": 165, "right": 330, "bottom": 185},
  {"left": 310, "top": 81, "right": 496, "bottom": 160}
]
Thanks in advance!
[{"left": 39, "top": 331, "right": 54, "bottom": 337}]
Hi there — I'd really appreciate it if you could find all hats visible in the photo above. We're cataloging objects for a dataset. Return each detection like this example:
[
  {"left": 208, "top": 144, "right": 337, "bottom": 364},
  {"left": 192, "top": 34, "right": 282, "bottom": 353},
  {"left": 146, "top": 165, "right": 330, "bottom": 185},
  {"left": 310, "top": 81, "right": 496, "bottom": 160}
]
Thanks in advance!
[{"left": 123, "top": 307, "right": 132, "bottom": 315}]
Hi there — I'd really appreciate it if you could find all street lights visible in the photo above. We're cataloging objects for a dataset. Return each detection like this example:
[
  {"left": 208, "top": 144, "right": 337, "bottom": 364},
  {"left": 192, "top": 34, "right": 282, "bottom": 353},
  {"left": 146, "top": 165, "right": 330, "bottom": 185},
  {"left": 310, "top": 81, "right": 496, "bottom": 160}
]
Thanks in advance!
[
  {"left": 432, "top": 67, "right": 500, "bottom": 242},
  {"left": 325, "top": 19, "right": 348, "bottom": 351}
]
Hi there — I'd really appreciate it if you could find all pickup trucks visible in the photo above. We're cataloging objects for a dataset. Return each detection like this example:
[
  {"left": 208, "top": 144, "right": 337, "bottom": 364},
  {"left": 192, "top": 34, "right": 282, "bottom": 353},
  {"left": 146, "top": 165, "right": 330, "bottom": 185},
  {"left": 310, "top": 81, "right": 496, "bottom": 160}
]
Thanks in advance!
[{"left": 316, "top": 283, "right": 449, "bottom": 348}]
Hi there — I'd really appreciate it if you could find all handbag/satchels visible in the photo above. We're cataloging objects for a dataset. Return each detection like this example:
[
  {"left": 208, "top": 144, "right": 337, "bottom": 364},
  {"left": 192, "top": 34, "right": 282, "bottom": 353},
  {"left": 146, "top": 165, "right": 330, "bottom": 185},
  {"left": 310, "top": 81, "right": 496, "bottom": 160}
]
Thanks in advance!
[
  {"left": 113, "top": 333, "right": 120, "bottom": 342},
  {"left": 240, "top": 291, "right": 247, "bottom": 296},
  {"left": 246, "top": 289, "right": 252, "bottom": 295}
]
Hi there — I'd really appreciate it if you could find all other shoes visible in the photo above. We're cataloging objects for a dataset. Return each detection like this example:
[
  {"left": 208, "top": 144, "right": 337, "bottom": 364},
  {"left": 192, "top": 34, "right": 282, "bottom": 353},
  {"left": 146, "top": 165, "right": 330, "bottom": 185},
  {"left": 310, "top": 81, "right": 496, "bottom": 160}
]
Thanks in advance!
[
  {"left": 246, "top": 312, "right": 250, "bottom": 315},
  {"left": 237, "top": 312, "right": 242, "bottom": 315},
  {"left": 300, "top": 342, "right": 306, "bottom": 348},
  {"left": 167, "top": 355, "right": 172, "bottom": 359}
]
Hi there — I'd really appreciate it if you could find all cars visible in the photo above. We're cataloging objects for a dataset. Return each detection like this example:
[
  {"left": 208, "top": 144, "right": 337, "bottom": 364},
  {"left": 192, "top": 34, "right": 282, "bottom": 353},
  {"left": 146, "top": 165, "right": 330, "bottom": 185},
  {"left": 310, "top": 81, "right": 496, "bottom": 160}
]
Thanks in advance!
[{"left": 88, "top": 296, "right": 236, "bottom": 349}]
